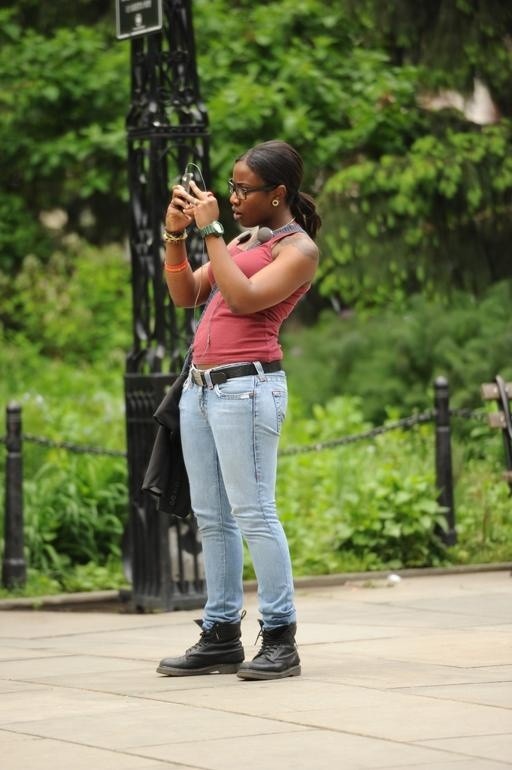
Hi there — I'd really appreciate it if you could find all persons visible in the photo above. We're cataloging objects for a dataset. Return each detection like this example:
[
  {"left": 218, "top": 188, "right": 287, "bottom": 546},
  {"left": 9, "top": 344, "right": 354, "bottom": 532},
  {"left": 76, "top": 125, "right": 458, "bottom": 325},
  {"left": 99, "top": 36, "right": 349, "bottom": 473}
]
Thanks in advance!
[{"left": 157, "top": 138, "right": 323, "bottom": 683}]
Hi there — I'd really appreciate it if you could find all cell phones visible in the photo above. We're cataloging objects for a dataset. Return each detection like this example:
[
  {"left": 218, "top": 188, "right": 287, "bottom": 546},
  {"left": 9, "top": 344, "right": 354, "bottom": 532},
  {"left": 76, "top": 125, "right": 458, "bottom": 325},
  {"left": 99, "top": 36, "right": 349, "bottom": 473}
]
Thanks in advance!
[{"left": 181, "top": 173, "right": 193, "bottom": 209}]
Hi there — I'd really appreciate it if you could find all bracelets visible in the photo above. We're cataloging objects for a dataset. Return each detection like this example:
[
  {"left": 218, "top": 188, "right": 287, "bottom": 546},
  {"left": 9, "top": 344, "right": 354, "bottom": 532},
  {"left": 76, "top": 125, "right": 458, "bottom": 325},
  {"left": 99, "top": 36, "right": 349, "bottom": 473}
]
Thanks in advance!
[
  {"left": 163, "top": 258, "right": 189, "bottom": 272},
  {"left": 162, "top": 229, "right": 188, "bottom": 242}
]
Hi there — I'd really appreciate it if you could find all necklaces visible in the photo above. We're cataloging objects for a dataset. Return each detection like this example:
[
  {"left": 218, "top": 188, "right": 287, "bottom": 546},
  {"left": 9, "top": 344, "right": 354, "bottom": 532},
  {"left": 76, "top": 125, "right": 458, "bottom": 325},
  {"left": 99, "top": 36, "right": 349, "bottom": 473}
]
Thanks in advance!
[{"left": 272, "top": 216, "right": 295, "bottom": 233}]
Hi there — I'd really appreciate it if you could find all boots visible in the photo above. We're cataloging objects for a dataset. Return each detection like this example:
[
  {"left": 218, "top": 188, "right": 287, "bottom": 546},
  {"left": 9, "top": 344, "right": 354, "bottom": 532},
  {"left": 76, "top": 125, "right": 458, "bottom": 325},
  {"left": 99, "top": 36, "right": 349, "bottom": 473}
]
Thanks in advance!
[
  {"left": 156, "top": 618, "right": 244, "bottom": 675},
  {"left": 238, "top": 619, "right": 301, "bottom": 681}
]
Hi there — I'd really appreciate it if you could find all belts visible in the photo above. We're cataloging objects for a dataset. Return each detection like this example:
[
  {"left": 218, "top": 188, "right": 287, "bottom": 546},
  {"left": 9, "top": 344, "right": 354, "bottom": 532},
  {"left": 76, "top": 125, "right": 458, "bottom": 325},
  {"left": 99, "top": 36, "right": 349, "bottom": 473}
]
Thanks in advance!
[{"left": 190, "top": 358, "right": 284, "bottom": 389}]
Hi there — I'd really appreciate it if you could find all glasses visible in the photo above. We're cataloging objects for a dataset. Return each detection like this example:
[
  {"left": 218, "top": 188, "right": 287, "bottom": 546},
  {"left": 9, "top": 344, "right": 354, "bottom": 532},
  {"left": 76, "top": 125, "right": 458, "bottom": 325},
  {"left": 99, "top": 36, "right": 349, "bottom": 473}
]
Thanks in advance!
[{"left": 227, "top": 179, "right": 277, "bottom": 199}]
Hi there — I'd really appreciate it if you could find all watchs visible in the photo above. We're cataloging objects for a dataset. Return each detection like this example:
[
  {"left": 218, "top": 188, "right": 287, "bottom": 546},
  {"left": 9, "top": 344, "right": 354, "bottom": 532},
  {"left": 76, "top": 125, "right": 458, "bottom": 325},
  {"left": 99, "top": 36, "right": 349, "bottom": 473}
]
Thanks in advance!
[{"left": 200, "top": 221, "right": 225, "bottom": 241}]
